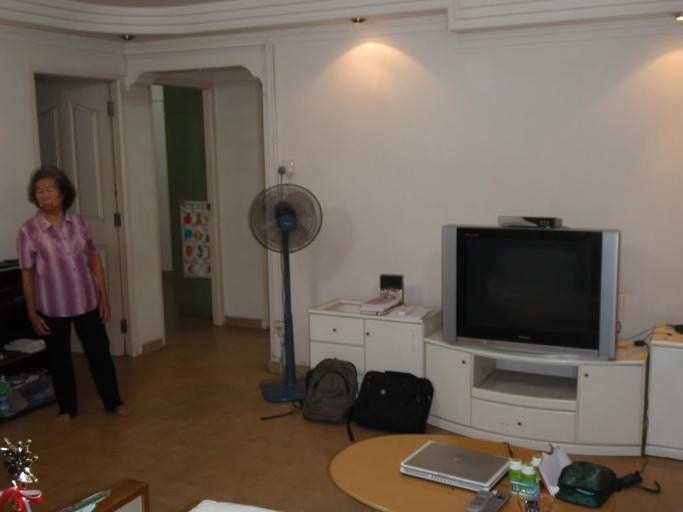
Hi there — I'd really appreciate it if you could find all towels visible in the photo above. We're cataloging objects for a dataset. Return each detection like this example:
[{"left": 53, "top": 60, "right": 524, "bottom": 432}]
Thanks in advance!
[{"left": 3, "top": 336, "right": 46, "bottom": 355}]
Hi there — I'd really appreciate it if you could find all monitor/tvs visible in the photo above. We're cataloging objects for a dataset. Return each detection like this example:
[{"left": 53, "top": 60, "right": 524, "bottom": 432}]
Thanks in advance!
[{"left": 442, "top": 225, "right": 620, "bottom": 360}]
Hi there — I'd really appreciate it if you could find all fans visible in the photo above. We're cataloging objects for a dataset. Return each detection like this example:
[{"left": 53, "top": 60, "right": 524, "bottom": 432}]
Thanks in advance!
[{"left": 248, "top": 182, "right": 323, "bottom": 404}]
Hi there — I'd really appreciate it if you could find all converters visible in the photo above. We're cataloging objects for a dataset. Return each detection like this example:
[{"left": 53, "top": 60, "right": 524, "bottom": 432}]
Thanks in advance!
[{"left": 619, "top": 473, "right": 643, "bottom": 488}]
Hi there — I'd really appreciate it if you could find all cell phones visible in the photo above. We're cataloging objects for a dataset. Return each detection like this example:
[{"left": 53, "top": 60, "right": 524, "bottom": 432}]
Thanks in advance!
[{"left": 527, "top": 500, "right": 538, "bottom": 512}]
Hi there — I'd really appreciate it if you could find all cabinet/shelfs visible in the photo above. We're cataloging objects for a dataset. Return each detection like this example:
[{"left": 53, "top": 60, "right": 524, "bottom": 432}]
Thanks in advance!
[
  {"left": 310, "top": 313, "right": 363, "bottom": 389},
  {"left": 471, "top": 398, "right": 577, "bottom": 445},
  {"left": 426, "top": 344, "right": 471, "bottom": 425},
  {"left": 1, "top": 259, "right": 57, "bottom": 421},
  {"left": 363, "top": 320, "right": 424, "bottom": 379},
  {"left": 579, "top": 364, "right": 641, "bottom": 447}
]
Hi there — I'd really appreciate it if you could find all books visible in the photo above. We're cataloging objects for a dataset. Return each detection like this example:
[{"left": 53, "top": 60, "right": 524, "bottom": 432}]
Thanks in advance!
[
  {"left": 616, "top": 345, "right": 649, "bottom": 364},
  {"left": 650, "top": 323, "right": 683, "bottom": 347},
  {"left": 361, "top": 298, "right": 431, "bottom": 319}
]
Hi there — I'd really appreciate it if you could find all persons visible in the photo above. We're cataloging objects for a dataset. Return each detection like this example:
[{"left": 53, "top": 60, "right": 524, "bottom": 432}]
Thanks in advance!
[{"left": 17, "top": 165, "right": 130, "bottom": 424}]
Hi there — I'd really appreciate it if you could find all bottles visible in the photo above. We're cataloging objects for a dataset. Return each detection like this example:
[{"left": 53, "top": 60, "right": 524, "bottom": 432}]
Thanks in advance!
[
  {"left": 0, "top": 376, "right": 9, "bottom": 416},
  {"left": 508, "top": 454, "right": 545, "bottom": 500}
]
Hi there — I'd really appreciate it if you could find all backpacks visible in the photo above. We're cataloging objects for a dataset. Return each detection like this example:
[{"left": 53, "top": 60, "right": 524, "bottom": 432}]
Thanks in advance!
[{"left": 302, "top": 358, "right": 358, "bottom": 424}]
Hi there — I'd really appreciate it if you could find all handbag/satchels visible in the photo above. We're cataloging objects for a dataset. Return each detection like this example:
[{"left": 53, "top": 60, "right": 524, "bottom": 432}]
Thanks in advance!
[{"left": 351, "top": 370, "right": 434, "bottom": 434}]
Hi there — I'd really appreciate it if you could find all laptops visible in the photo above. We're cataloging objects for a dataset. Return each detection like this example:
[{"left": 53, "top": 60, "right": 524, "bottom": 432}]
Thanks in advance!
[{"left": 400, "top": 440, "right": 511, "bottom": 493}]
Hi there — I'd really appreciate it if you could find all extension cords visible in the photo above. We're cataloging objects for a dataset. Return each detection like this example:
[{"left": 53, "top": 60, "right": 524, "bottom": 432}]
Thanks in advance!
[{"left": 616, "top": 342, "right": 648, "bottom": 356}]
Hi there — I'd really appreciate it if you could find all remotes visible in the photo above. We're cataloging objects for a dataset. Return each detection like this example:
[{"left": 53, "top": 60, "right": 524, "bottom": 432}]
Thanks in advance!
[
  {"left": 480, "top": 491, "right": 510, "bottom": 512},
  {"left": 466, "top": 490, "right": 492, "bottom": 512}
]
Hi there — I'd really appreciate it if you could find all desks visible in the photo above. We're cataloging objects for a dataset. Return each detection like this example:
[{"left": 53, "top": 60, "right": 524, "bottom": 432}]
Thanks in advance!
[{"left": 328, "top": 433, "right": 682, "bottom": 512}]
